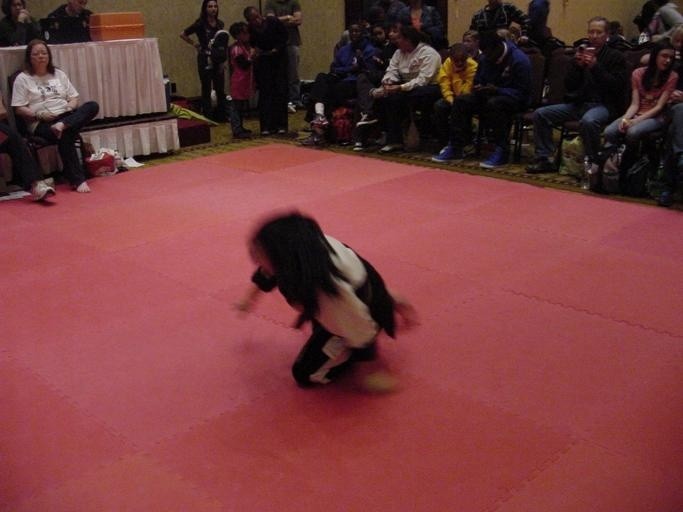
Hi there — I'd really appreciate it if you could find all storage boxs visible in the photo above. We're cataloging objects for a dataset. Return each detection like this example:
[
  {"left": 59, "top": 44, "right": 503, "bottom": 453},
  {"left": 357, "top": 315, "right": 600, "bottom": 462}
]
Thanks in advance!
[{"left": 87, "top": 10, "right": 146, "bottom": 42}]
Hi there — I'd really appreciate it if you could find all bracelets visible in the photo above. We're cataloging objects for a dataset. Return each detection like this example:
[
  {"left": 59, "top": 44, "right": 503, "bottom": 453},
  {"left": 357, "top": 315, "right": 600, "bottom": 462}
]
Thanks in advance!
[
  {"left": 287, "top": 14, "right": 289, "bottom": 20},
  {"left": 32, "top": 111, "right": 43, "bottom": 120}
]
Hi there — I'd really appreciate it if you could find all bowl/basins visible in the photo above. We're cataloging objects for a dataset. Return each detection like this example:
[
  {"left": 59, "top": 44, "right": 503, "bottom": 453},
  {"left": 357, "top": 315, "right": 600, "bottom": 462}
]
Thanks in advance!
[{"left": 86, "top": 157, "right": 113, "bottom": 176}]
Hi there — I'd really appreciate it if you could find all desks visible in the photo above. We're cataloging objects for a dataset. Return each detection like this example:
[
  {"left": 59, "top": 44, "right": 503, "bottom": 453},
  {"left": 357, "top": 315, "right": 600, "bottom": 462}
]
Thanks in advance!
[{"left": 0, "top": 35, "right": 159, "bottom": 126}]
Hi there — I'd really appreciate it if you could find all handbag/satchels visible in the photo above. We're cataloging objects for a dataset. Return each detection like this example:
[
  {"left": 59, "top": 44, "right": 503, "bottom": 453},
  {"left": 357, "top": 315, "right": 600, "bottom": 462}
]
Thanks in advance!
[
  {"left": 590, "top": 141, "right": 649, "bottom": 193},
  {"left": 325, "top": 106, "right": 354, "bottom": 142}
]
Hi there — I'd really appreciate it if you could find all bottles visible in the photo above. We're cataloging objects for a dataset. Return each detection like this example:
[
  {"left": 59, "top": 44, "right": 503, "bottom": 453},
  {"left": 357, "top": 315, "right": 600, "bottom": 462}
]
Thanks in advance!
[
  {"left": 163, "top": 74, "right": 170, "bottom": 111},
  {"left": 579, "top": 156, "right": 590, "bottom": 190},
  {"left": 113, "top": 143, "right": 123, "bottom": 173}
]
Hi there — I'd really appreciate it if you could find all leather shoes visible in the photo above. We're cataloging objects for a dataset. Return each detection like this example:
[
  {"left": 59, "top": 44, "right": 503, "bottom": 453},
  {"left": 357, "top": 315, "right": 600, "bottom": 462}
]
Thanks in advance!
[
  {"left": 659, "top": 191, "right": 673, "bottom": 205},
  {"left": 676, "top": 158, "right": 683, "bottom": 171}
]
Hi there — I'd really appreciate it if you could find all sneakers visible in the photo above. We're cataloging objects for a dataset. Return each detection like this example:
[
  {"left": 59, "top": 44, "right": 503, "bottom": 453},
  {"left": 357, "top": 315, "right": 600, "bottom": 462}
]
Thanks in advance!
[
  {"left": 462, "top": 143, "right": 477, "bottom": 158},
  {"left": 479, "top": 145, "right": 510, "bottom": 169},
  {"left": 431, "top": 145, "right": 464, "bottom": 164},
  {"left": 356, "top": 112, "right": 379, "bottom": 129},
  {"left": 30, "top": 180, "right": 56, "bottom": 201},
  {"left": 232, "top": 126, "right": 297, "bottom": 138},
  {"left": 310, "top": 113, "right": 331, "bottom": 129},
  {"left": 301, "top": 130, "right": 327, "bottom": 146},
  {"left": 288, "top": 102, "right": 296, "bottom": 113},
  {"left": 525, "top": 159, "right": 552, "bottom": 173},
  {"left": 378, "top": 143, "right": 405, "bottom": 155},
  {"left": 353, "top": 139, "right": 368, "bottom": 152}
]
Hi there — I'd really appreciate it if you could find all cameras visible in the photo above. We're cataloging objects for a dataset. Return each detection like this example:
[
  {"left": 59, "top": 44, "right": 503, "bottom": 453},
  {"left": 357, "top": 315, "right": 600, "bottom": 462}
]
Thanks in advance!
[{"left": 578, "top": 44, "right": 596, "bottom": 54}]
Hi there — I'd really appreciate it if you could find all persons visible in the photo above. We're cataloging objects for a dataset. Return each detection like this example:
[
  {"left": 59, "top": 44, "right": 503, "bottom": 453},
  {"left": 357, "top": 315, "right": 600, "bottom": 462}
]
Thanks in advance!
[
  {"left": 0, "top": 0, "right": 40, "bottom": 47},
  {"left": 243, "top": 6, "right": 289, "bottom": 135},
  {"left": 297, "top": 0, "right": 681, "bottom": 205},
  {"left": 264, "top": 0, "right": 304, "bottom": 114},
  {"left": 227, "top": 21, "right": 255, "bottom": 137},
  {"left": 10, "top": 39, "right": 99, "bottom": 192},
  {"left": 178, "top": 0, "right": 227, "bottom": 123},
  {"left": 0, "top": 95, "right": 56, "bottom": 201},
  {"left": 230, "top": 212, "right": 418, "bottom": 395},
  {"left": 46, "top": 0, "right": 93, "bottom": 43}
]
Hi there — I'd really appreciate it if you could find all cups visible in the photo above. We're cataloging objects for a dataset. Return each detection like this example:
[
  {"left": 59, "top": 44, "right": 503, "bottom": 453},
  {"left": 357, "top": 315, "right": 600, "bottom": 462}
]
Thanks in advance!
[{"left": 40, "top": 18, "right": 54, "bottom": 43}]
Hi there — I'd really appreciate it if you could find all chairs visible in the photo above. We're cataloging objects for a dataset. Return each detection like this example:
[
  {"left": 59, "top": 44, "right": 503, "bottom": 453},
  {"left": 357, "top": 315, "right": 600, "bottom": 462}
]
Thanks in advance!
[
  {"left": 7, "top": 68, "right": 89, "bottom": 180},
  {"left": 437, "top": 36, "right": 653, "bottom": 170}
]
